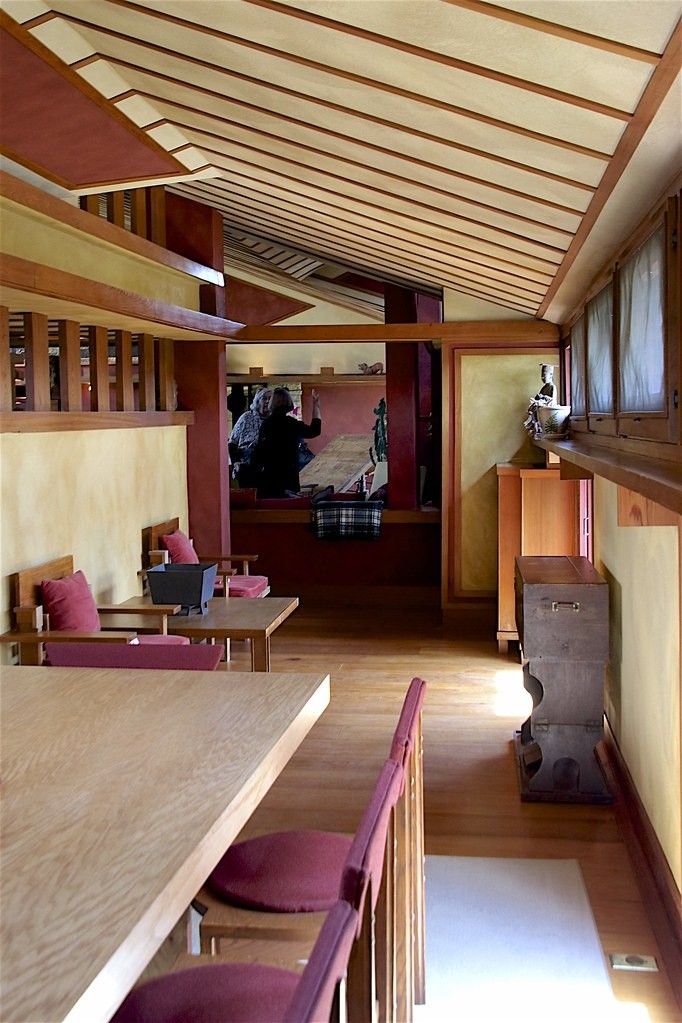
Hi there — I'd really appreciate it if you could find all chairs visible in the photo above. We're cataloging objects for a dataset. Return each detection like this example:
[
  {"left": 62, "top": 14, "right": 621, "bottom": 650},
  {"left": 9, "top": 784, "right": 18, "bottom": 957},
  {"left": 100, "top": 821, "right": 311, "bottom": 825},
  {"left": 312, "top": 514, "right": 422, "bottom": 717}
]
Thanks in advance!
[
  {"left": 0, "top": 555, "right": 191, "bottom": 671},
  {"left": 138, "top": 515, "right": 269, "bottom": 664},
  {"left": 44, "top": 641, "right": 226, "bottom": 671},
  {"left": 110, "top": 677, "right": 428, "bottom": 1023}
]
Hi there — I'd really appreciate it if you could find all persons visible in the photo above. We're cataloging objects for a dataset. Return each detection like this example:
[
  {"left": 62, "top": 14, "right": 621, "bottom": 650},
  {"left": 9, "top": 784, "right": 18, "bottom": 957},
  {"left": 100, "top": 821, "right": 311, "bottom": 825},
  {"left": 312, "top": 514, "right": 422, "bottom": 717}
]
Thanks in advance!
[
  {"left": 524, "top": 363, "right": 559, "bottom": 437},
  {"left": 228, "top": 387, "right": 321, "bottom": 499}
]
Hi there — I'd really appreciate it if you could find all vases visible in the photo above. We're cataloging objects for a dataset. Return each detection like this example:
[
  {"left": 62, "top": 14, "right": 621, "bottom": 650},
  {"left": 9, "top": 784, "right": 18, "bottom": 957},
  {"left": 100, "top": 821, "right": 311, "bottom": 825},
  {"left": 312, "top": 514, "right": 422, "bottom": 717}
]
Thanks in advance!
[{"left": 537, "top": 406, "right": 571, "bottom": 439}]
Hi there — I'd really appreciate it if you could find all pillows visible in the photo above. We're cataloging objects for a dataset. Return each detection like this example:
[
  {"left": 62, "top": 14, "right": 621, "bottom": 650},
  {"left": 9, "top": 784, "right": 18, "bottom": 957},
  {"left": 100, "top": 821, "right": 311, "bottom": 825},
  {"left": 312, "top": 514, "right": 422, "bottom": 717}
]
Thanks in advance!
[
  {"left": 161, "top": 530, "right": 201, "bottom": 564},
  {"left": 41, "top": 570, "right": 101, "bottom": 633}
]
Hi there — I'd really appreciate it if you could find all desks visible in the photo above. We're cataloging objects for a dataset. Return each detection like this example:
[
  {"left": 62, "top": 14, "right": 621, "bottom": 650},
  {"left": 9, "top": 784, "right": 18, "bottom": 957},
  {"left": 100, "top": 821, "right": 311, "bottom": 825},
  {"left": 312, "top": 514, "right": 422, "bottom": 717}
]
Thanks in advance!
[
  {"left": 98, "top": 595, "right": 299, "bottom": 672},
  {"left": 0, "top": 663, "right": 330, "bottom": 1023}
]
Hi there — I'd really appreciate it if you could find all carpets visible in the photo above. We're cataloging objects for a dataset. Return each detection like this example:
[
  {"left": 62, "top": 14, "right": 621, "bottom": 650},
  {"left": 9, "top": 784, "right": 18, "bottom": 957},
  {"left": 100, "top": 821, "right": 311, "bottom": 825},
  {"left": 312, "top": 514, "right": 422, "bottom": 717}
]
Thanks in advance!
[{"left": 340, "top": 852, "right": 619, "bottom": 1023}]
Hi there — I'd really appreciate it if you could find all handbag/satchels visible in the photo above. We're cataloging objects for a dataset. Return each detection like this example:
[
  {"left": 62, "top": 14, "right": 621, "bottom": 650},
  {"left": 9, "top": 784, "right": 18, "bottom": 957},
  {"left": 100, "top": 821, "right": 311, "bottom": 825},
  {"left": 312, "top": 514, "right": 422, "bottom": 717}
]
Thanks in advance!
[{"left": 238, "top": 462, "right": 255, "bottom": 488}]
